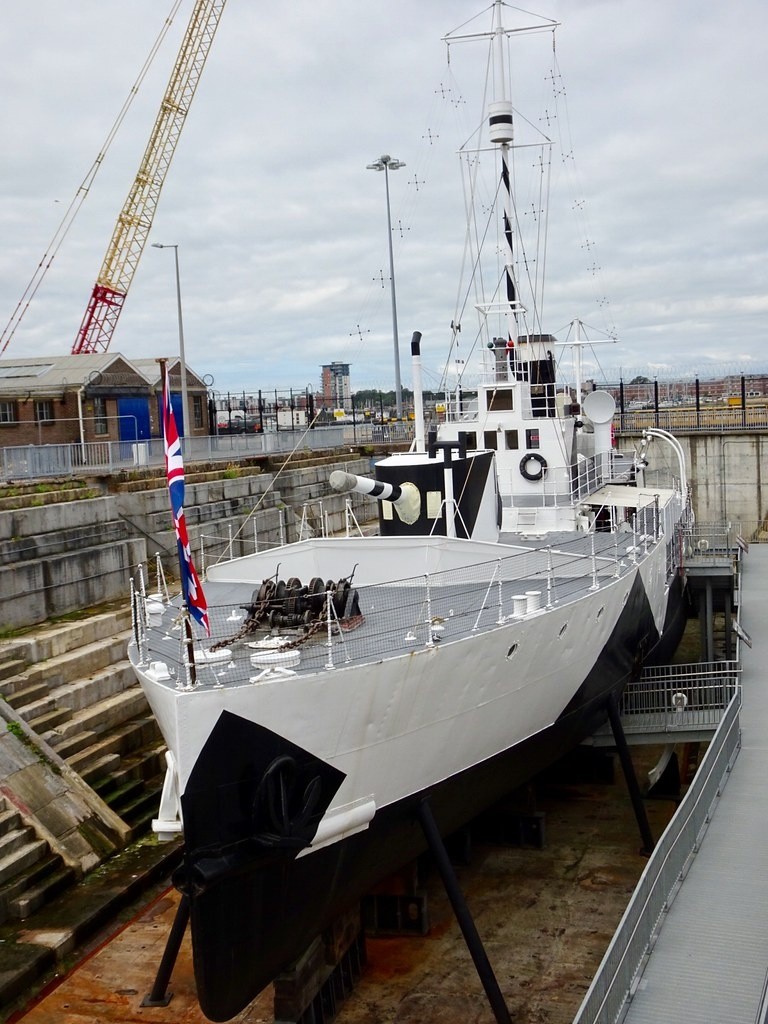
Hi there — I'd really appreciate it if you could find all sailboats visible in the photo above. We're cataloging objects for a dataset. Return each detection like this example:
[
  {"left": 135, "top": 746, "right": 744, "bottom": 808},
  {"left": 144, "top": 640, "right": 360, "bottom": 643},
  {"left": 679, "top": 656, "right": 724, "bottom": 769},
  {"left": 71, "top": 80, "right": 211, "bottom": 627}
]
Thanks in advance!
[{"left": 123, "top": 0, "right": 695, "bottom": 1024}]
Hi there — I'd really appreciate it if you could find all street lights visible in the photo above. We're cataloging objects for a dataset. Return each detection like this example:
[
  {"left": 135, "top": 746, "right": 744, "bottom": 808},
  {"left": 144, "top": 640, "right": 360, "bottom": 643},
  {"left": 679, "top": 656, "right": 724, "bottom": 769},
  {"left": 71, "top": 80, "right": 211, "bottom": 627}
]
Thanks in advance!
[
  {"left": 151, "top": 242, "right": 192, "bottom": 462},
  {"left": 365, "top": 154, "right": 408, "bottom": 435}
]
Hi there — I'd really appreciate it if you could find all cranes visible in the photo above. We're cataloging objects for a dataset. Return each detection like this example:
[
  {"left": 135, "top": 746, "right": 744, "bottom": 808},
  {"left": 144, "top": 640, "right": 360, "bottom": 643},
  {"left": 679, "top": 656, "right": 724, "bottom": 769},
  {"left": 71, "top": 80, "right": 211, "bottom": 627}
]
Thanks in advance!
[{"left": 1, "top": 1, "right": 228, "bottom": 358}]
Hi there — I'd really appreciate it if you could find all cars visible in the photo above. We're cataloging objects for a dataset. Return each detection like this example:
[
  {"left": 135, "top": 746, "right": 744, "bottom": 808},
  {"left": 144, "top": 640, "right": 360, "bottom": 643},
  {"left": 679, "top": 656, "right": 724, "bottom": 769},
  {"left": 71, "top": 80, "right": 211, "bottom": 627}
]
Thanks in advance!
[
  {"left": 628, "top": 391, "right": 765, "bottom": 410},
  {"left": 218, "top": 415, "right": 262, "bottom": 434}
]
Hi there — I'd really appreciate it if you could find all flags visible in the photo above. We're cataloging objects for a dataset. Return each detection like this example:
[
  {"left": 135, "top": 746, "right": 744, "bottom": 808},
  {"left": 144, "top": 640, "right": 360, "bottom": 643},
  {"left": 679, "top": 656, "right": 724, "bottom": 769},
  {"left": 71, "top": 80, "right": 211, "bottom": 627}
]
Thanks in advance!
[{"left": 161, "top": 360, "right": 210, "bottom": 638}]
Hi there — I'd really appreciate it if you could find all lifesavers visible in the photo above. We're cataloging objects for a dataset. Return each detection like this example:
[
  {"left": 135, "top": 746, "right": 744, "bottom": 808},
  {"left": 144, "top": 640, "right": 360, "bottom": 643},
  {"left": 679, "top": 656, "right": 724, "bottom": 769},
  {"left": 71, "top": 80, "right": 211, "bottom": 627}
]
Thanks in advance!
[{"left": 517, "top": 454, "right": 547, "bottom": 482}]
